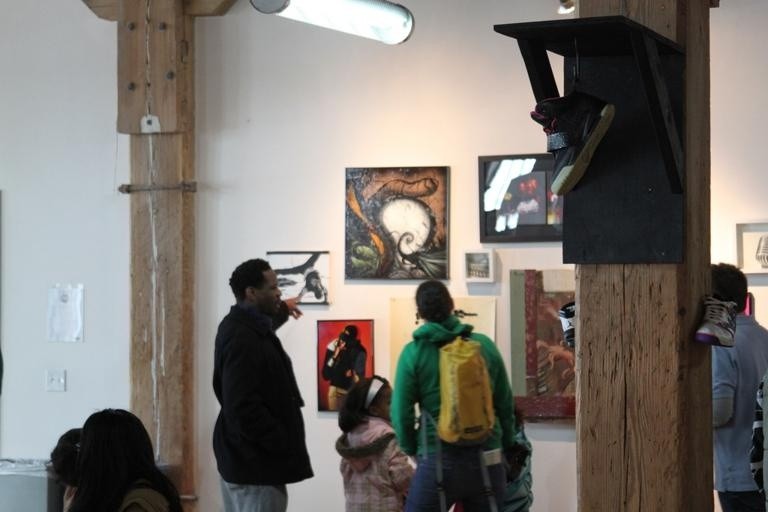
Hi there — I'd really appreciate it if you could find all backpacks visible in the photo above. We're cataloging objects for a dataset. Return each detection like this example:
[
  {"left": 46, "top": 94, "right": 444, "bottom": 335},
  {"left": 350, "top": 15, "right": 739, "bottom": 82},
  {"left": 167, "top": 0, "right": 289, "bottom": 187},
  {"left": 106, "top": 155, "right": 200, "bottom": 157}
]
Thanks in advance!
[{"left": 412, "top": 332, "right": 496, "bottom": 451}]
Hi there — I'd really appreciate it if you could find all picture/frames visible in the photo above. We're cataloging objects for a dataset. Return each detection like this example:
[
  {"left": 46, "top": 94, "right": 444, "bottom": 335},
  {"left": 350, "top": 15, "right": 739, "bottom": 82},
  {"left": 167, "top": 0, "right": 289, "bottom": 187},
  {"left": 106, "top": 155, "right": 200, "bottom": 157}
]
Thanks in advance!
[
  {"left": 316, "top": 319, "right": 374, "bottom": 413},
  {"left": 462, "top": 248, "right": 495, "bottom": 284},
  {"left": 732, "top": 218, "right": 768, "bottom": 275},
  {"left": 478, "top": 153, "right": 565, "bottom": 244}
]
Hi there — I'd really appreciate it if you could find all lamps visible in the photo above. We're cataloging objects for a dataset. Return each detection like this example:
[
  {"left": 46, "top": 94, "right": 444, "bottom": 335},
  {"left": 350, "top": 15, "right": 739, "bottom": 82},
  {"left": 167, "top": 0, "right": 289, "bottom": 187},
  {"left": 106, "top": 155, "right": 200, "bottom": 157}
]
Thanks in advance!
[{"left": 249, "top": 0, "right": 413, "bottom": 45}]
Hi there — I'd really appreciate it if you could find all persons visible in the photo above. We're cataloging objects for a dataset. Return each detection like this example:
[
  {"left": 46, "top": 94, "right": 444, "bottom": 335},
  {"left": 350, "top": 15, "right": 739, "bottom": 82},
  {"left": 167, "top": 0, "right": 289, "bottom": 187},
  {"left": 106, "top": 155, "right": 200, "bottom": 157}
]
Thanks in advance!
[
  {"left": 213, "top": 259, "right": 314, "bottom": 512},
  {"left": 335, "top": 375, "right": 415, "bottom": 512},
  {"left": 50, "top": 428, "right": 84, "bottom": 512},
  {"left": 388, "top": 280, "right": 517, "bottom": 512},
  {"left": 712, "top": 262, "right": 768, "bottom": 512},
  {"left": 344, "top": 325, "right": 366, "bottom": 383},
  {"left": 503, "top": 405, "right": 534, "bottom": 512},
  {"left": 749, "top": 372, "right": 767, "bottom": 511},
  {"left": 322, "top": 332, "right": 355, "bottom": 410},
  {"left": 67, "top": 407, "right": 183, "bottom": 512}
]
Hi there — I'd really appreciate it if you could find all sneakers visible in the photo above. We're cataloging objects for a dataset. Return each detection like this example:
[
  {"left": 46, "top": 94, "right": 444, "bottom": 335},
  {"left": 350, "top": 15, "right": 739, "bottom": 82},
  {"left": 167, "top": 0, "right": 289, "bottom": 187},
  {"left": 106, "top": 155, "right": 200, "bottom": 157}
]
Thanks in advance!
[
  {"left": 558, "top": 301, "right": 576, "bottom": 348},
  {"left": 695, "top": 296, "right": 738, "bottom": 348}
]
